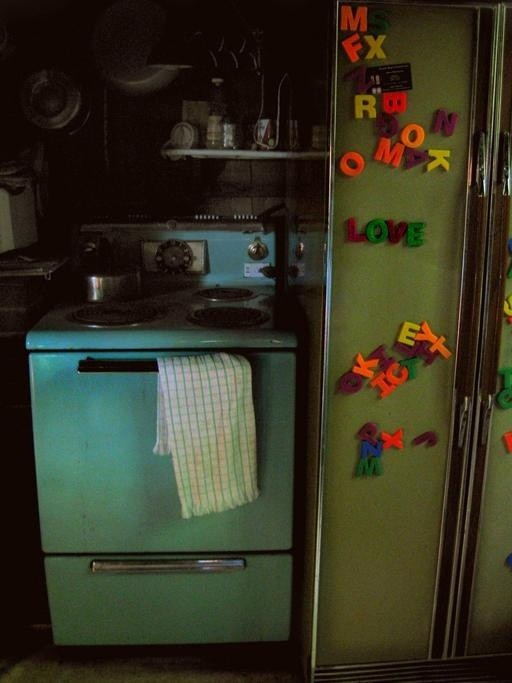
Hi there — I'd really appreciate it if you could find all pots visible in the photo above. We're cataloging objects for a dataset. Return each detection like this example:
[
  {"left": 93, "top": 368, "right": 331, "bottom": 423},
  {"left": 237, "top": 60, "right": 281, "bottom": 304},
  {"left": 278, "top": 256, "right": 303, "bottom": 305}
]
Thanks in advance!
[{"left": 84, "top": 239, "right": 139, "bottom": 305}]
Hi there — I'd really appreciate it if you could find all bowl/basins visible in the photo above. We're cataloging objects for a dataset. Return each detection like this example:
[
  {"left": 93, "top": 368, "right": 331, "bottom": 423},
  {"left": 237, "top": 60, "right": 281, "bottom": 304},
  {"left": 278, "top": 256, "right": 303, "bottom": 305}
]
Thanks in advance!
[
  {"left": 20, "top": 61, "right": 90, "bottom": 136},
  {"left": 88, "top": 3, "right": 179, "bottom": 94}
]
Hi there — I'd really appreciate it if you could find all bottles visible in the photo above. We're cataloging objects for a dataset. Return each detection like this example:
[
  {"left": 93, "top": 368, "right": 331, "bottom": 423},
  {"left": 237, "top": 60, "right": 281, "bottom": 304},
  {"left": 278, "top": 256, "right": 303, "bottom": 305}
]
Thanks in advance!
[{"left": 205, "top": 96, "right": 241, "bottom": 149}]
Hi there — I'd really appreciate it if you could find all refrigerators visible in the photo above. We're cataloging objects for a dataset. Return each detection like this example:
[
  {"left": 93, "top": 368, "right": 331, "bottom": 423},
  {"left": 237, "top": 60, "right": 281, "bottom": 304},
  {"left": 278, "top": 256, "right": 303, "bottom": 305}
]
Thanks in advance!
[{"left": 315, "top": 4, "right": 512, "bottom": 668}]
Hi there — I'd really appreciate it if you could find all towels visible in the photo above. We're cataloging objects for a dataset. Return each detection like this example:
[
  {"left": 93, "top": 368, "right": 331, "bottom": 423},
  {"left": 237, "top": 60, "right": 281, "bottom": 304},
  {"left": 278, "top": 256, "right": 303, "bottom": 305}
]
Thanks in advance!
[{"left": 147, "top": 353, "right": 261, "bottom": 518}]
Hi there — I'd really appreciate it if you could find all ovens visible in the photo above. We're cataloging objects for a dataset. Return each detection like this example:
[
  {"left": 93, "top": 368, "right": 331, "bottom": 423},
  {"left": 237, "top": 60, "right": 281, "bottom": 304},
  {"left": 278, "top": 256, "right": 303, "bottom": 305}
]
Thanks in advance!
[{"left": 26, "top": 351, "right": 291, "bottom": 648}]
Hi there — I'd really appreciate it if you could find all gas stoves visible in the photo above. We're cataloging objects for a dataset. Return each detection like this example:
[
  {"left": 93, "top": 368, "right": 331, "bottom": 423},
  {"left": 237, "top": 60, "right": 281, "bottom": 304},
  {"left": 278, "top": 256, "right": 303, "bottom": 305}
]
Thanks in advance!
[{"left": 28, "top": 267, "right": 296, "bottom": 332}]
